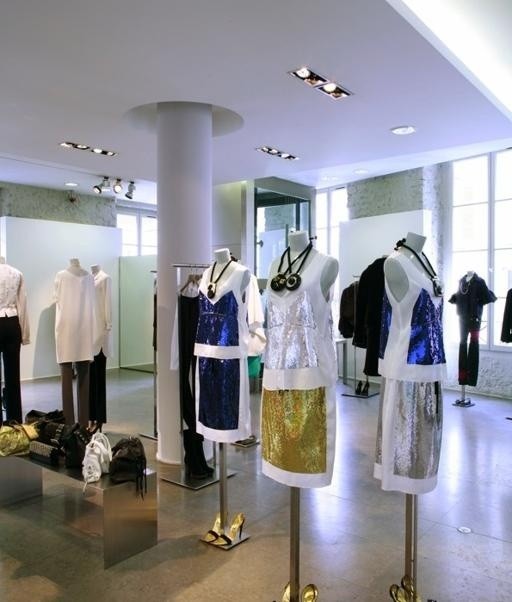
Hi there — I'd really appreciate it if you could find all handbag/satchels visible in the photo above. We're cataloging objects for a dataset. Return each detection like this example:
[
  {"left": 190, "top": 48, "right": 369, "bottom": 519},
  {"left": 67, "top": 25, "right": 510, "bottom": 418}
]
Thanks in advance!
[{"left": 1, "top": 409, "right": 148, "bottom": 484}]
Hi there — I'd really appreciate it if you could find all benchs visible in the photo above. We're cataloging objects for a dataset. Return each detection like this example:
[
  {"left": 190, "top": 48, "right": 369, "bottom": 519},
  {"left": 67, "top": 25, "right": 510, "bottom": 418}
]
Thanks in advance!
[{"left": 0, "top": 449, "right": 156, "bottom": 571}]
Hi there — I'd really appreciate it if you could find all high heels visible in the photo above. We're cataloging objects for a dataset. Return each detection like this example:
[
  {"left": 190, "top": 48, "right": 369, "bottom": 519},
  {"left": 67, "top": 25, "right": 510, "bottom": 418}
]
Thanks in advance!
[
  {"left": 201, "top": 510, "right": 246, "bottom": 546},
  {"left": 235, "top": 435, "right": 259, "bottom": 446},
  {"left": 355, "top": 381, "right": 369, "bottom": 396},
  {"left": 389, "top": 575, "right": 422, "bottom": 602},
  {"left": 451, "top": 398, "right": 473, "bottom": 407},
  {"left": 280, "top": 580, "right": 319, "bottom": 601}
]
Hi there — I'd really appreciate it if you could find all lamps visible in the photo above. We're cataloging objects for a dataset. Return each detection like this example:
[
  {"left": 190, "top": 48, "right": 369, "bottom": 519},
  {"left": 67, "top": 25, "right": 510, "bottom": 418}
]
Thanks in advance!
[
  {"left": 113, "top": 179, "right": 123, "bottom": 194},
  {"left": 93, "top": 177, "right": 112, "bottom": 194},
  {"left": 125, "top": 181, "right": 136, "bottom": 199}
]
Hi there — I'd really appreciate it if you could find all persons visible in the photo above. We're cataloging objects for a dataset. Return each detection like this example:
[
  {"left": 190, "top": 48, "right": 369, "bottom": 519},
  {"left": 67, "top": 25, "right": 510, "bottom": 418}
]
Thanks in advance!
[
  {"left": 379, "top": 232, "right": 449, "bottom": 497},
  {"left": 0, "top": 256, "right": 30, "bottom": 425},
  {"left": 53, "top": 258, "right": 111, "bottom": 431},
  {"left": 264, "top": 230, "right": 349, "bottom": 488},
  {"left": 187, "top": 248, "right": 266, "bottom": 442},
  {"left": 449, "top": 270, "right": 497, "bottom": 388}
]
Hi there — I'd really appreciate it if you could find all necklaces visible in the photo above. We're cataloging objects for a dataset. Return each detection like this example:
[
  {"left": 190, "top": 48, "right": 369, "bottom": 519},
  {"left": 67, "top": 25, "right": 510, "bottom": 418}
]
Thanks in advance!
[
  {"left": 460, "top": 282, "right": 470, "bottom": 296},
  {"left": 207, "top": 255, "right": 237, "bottom": 298},
  {"left": 271, "top": 237, "right": 318, "bottom": 290},
  {"left": 395, "top": 237, "right": 443, "bottom": 298}
]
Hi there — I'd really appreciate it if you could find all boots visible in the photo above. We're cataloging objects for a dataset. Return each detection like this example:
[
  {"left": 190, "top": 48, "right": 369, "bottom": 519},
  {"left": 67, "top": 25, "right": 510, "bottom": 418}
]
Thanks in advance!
[{"left": 182, "top": 429, "right": 215, "bottom": 478}]
